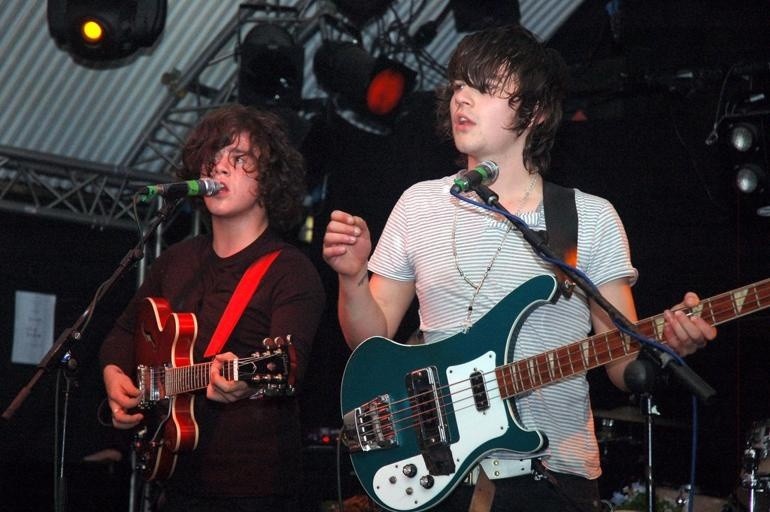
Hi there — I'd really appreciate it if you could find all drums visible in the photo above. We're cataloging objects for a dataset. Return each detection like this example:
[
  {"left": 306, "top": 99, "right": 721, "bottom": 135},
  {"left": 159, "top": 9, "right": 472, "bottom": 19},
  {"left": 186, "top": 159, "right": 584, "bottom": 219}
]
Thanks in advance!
[
  {"left": 596, "top": 435, "right": 645, "bottom": 500},
  {"left": 734, "top": 419, "right": 770, "bottom": 511},
  {"left": 654, "top": 487, "right": 731, "bottom": 512}
]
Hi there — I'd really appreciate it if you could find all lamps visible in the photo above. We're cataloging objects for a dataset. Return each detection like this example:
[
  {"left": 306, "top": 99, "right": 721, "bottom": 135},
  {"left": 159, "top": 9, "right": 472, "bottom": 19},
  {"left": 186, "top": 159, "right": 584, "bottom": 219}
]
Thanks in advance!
[{"left": 238, "top": 0, "right": 520, "bottom": 120}]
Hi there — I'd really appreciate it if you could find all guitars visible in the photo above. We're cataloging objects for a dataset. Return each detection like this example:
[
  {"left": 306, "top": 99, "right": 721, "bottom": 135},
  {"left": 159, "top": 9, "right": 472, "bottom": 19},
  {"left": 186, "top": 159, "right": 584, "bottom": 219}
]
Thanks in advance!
[
  {"left": 134, "top": 297, "right": 299, "bottom": 484},
  {"left": 339, "top": 273, "right": 770, "bottom": 512}
]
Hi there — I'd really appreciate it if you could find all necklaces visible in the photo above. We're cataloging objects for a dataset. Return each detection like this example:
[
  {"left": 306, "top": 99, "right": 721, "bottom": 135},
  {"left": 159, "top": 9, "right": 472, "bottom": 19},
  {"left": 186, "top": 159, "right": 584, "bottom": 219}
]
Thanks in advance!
[{"left": 448, "top": 171, "right": 537, "bottom": 334}]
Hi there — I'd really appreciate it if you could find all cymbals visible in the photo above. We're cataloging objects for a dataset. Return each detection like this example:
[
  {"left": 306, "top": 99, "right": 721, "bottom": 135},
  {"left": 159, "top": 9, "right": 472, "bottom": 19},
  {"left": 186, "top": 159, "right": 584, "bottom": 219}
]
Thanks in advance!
[{"left": 592, "top": 404, "right": 666, "bottom": 427}]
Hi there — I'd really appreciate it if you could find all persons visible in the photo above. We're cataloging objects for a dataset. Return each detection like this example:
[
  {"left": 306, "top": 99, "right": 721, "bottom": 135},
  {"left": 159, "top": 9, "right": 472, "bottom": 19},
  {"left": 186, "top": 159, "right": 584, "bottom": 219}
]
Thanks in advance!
[
  {"left": 94, "top": 106, "right": 330, "bottom": 510},
  {"left": 317, "top": 27, "right": 724, "bottom": 510}
]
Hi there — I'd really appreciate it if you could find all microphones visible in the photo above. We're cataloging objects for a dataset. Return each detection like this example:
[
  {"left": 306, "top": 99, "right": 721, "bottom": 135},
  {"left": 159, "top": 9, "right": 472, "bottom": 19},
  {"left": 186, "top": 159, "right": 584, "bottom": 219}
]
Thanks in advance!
[
  {"left": 451, "top": 160, "right": 500, "bottom": 194},
  {"left": 141, "top": 176, "right": 222, "bottom": 200}
]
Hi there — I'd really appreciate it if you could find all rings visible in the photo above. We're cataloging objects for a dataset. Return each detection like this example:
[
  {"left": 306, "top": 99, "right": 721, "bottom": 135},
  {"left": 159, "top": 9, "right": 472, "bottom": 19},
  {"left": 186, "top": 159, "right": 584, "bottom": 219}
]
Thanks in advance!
[{"left": 112, "top": 405, "right": 124, "bottom": 415}]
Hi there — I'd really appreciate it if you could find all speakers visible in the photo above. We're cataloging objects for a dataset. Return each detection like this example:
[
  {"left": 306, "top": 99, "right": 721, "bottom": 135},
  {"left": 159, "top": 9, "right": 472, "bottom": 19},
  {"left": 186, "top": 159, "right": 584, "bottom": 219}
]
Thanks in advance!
[{"left": 719, "top": 107, "right": 768, "bottom": 209}]
